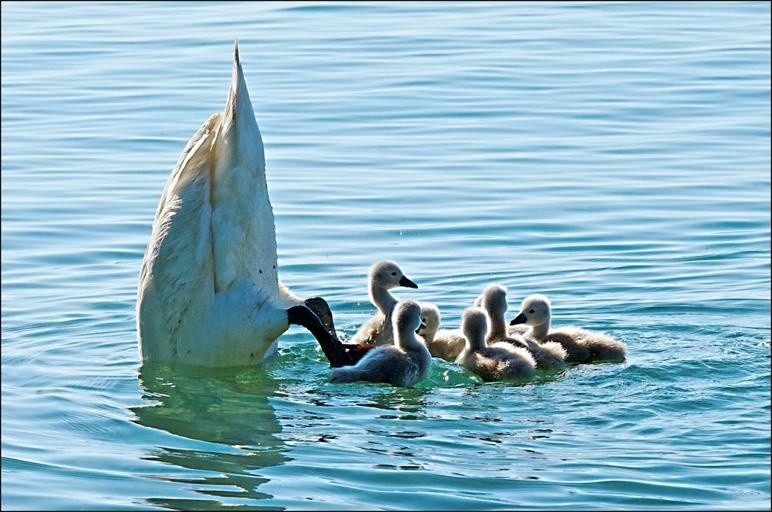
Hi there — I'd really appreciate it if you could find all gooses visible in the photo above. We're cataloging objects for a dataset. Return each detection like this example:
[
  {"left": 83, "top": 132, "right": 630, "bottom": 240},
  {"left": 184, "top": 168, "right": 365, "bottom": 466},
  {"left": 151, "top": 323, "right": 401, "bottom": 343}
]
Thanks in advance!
[
  {"left": 134, "top": 37, "right": 366, "bottom": 372},
  {"left": 331, "top": 260, "right": 627, "bottom": 386}
]
